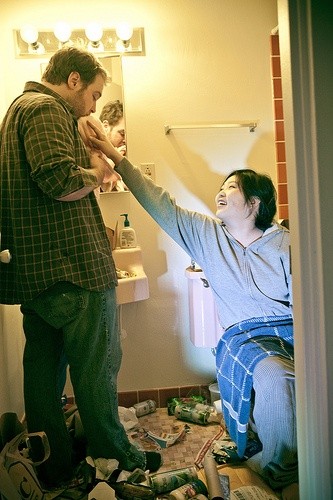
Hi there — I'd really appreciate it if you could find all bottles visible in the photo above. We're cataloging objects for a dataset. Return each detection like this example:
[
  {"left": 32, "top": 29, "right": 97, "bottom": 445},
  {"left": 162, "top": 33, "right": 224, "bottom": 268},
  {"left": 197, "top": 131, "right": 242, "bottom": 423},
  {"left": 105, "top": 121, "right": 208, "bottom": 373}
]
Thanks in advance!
[{"left": 93, "top": 478, "right": 157, "bottom": 500}]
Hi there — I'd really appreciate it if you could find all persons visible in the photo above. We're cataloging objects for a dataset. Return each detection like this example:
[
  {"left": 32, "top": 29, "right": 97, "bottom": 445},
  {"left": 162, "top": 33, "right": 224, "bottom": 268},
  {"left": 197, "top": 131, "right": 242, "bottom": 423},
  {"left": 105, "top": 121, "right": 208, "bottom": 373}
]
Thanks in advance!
[
  {"left": 87, "top": 119, "right": 293, "bottom": 487},
  {"left": 0, "top": 45, "right": 161, "bottom": 491},
  {"left": 98, "top": 100, "right": 128, "bottom": 192}
]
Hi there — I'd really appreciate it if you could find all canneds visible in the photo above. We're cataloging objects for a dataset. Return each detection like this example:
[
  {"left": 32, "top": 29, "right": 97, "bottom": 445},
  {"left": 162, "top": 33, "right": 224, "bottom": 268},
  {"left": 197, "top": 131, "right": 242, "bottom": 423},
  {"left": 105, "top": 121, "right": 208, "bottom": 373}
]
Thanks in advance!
[
  {"left": 131, "top": 400, "right": 156, "bottom": 417},
  {"left": 115, "top": 466, "right": 209, "bottom": 500},
  {"left": 167, "top": 395, "right": 210, "bottom": 425}
]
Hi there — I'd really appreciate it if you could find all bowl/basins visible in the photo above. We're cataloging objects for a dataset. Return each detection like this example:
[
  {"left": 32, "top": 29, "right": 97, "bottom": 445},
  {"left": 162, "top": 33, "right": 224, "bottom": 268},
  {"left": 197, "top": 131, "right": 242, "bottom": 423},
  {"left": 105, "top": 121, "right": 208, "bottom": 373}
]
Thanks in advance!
[{"left": 209, "top": 383, "right": 220, "bottom": 405}]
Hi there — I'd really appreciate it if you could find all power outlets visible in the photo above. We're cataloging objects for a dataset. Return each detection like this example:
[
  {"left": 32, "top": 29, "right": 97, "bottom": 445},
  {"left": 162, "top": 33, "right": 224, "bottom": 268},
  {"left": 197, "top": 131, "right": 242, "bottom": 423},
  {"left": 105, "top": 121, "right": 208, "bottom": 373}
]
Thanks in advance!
[{"left": 139, "top": 163, "right": 155, "bottom": 182}]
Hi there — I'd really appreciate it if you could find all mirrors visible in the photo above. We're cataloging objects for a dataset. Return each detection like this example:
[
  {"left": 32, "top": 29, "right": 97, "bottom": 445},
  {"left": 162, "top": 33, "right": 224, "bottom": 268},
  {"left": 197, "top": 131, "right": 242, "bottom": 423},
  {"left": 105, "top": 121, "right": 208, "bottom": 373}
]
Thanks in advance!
[{"left": 90, "top": 54, "right": 129, "bottom": 196}]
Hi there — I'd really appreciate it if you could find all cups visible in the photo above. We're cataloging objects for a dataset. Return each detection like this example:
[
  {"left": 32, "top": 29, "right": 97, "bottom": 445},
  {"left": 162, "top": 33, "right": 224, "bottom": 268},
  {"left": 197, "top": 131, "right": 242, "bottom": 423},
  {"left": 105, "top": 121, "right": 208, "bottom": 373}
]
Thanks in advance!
[{"left": 213, "top": 399, "right": 222, "bottom": 414}]
